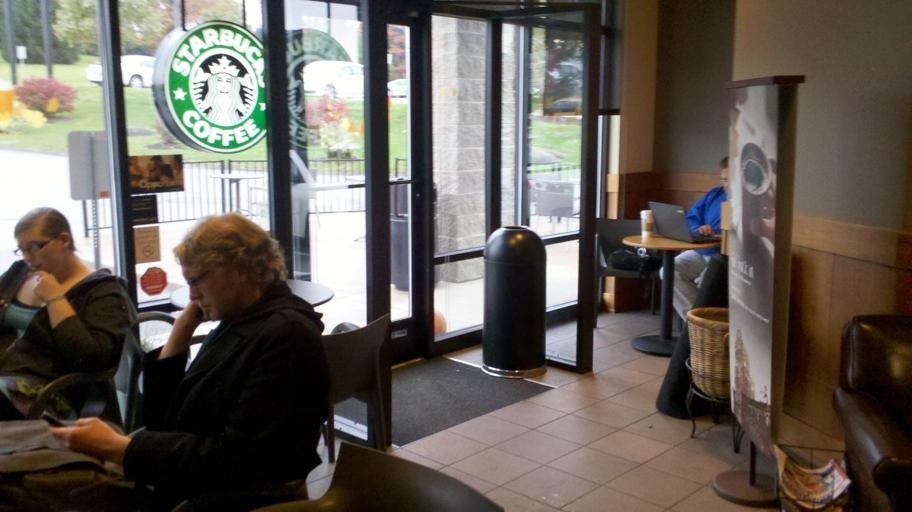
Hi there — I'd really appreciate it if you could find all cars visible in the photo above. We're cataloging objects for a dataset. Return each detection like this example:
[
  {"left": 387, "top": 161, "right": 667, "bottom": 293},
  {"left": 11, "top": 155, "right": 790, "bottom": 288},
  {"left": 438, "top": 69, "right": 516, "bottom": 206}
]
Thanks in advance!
[
  {"left": 387, "top": 77, "right": 408, "bottom": 98},
  {"left": 87, "top": 53, "right": 158, "bottom": 89},
  {"left": 542, "top": 97, "right": 581, "bottom": 115},
  {"left": 304, "top": 60, "right": 365, "bottom": 97}
]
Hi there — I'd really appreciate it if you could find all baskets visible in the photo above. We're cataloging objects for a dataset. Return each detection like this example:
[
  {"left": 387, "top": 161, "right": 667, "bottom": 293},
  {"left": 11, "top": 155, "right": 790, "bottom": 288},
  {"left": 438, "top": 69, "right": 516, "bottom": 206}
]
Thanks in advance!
[{"left": 684, "top": 306, "right": 732, "bottom": 401}]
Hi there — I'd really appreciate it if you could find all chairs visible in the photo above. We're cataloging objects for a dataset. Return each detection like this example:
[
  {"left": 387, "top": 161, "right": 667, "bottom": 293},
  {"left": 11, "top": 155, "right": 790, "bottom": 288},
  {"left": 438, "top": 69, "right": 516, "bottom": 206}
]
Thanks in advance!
[
  {"left": 588, "top": 215, "right": 663, "bottom": 333},
  {"left": 24, "top": 303, "right": 509, "bottom": 512},
  {"left": 826, "top": 309, "right": 910, "bottom": 512}
]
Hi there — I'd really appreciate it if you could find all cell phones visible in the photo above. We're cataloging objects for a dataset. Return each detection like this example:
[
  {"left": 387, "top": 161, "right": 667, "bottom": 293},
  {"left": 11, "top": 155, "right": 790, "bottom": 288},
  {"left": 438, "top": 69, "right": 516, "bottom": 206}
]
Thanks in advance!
[{"left": 43, "top": 409, "right": 69, "bottom": 431}]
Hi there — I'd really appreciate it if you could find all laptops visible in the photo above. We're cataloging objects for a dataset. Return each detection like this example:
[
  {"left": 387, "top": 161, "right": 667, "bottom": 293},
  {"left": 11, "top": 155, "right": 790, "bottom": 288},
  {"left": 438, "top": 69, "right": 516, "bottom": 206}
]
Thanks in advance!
[{"left": 647, "top": 201, "right": 722, "bottom": 243}]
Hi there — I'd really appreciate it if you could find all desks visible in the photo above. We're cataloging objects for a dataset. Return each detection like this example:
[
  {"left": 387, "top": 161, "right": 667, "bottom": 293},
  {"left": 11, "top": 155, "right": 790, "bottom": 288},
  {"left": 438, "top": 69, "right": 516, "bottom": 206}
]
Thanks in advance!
[
  {"left": 620, "top": 230, "right": 722, "bottom": 359},
  {"left": 167, "top": 274, "right": 336, "bottom": 311}
]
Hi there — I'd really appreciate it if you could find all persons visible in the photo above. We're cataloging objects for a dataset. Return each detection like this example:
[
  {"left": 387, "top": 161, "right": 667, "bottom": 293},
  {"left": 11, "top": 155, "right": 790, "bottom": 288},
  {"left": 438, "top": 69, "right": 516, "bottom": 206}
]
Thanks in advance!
[
  {"left": 0, "top": 205, "right": 131, "bottom": 431},
  {"left": 200, "top": 56, "right": 247, "bottom": 126},
  {"left": 48, "top": 213, "right": 326, "bottom": 510},
  {"left": 659, "top": 158, "right": 729, "bottom": 322}
]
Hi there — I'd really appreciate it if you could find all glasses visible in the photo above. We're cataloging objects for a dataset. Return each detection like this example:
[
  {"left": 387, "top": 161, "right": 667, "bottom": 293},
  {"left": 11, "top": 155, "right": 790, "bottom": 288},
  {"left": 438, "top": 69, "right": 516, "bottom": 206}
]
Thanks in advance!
[
  {"left": 12, "top": 236, "right": 60, "bottom": 258},
  {"left": 186, "top": 267, "right": 211, "bottom": 288}
]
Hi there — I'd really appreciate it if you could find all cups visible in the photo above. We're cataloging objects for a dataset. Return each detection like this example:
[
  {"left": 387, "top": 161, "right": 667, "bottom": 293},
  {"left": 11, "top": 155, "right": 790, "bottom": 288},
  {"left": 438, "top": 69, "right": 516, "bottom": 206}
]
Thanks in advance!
[{"left": 640, "top": 210, "right": 654, "bottom": 239}]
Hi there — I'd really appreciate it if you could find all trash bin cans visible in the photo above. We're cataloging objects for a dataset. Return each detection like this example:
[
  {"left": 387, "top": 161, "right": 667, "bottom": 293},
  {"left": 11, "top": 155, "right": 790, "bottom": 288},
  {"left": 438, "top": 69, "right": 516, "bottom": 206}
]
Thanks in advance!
[
  {"left": 389, "top": 178, "right": 409, "bottom": 290},
  {"left": 481, "top": 226, "right": 546, "bottom": 378}
]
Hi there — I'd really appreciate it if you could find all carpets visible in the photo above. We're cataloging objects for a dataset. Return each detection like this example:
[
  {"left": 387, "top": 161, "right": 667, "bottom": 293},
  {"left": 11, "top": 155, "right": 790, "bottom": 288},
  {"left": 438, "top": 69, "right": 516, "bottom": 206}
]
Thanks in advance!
[{"left": 325, "top": 350, "right": 558, "bottom": 452}]
